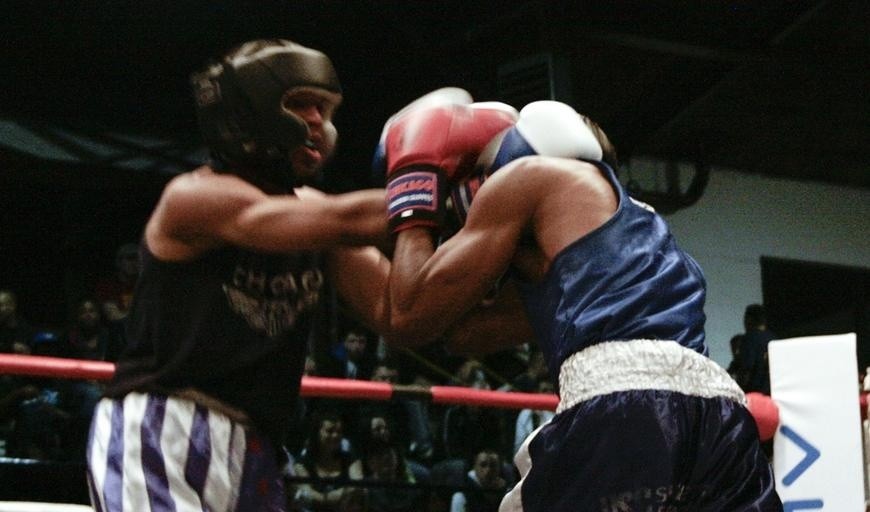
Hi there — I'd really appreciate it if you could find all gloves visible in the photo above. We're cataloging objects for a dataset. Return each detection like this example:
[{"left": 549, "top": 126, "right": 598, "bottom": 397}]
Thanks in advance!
[{"left": 387, "top": 89, "right": 517, "bottom": 232}]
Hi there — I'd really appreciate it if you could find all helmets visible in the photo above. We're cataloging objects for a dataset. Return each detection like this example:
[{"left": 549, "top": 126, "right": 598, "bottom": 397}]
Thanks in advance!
[
  {"left": 451, "top": 101, "right": 619, "bottom": 226},
  {"left": 193, "top": 42, "right": 340, "bottom": 189}
]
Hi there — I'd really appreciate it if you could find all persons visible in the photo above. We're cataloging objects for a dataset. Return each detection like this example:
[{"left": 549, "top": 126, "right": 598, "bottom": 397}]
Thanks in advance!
[
  {"left": 83, "top": 37, "right": 604, "bottom": 512},
  {"left": 293, "top": 86, "right": 784, "bottom": 512}
]
